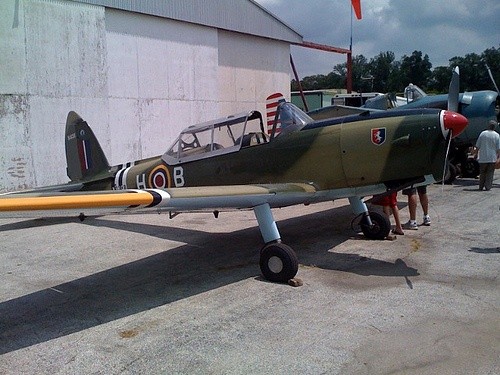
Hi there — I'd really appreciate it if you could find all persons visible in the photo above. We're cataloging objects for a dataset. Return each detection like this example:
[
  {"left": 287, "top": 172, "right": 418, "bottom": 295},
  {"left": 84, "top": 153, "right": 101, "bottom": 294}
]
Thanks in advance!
[
  {"left": 474, "top": 120, "right": 500, "bottom": 191},
  {"left": 401, "top": 184, "right": 432, "bottom": 230},
  {"left": 383, "top": 191, "right": 405, "bottom": 235}
]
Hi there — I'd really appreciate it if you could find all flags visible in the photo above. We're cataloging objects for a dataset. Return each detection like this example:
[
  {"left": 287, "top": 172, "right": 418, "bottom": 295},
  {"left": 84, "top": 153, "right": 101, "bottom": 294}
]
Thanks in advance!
[{"left": 351, "top": 0, "right": 362, "bottom": 20}]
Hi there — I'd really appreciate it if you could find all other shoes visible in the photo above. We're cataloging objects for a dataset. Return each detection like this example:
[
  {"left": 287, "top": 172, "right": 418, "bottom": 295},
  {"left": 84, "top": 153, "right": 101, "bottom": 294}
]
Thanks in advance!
[
  {"left": 386, "top": 234, "right": 394, "bottom": 240},
  {"left": 391, "top": 228, "right": 404, "bottom": 235}
]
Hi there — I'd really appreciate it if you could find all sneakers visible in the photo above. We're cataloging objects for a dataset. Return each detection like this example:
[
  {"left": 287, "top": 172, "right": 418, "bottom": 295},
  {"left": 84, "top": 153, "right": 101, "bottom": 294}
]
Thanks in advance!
[
  {"left": 423, "top": 217, "right": 431, "bottom": 224},
  {"left": 401, "top": 220, "right": 418, "bottom": 230}
]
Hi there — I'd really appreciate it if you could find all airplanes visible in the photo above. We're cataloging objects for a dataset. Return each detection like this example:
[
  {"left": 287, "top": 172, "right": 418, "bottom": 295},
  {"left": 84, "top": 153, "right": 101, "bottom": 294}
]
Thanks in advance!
[
  {"left": 0, "top": 65, "right": 470, "bottom": 284},
  {"left": 355, "top": 82, "right": 500, "bottom": 186}
]
